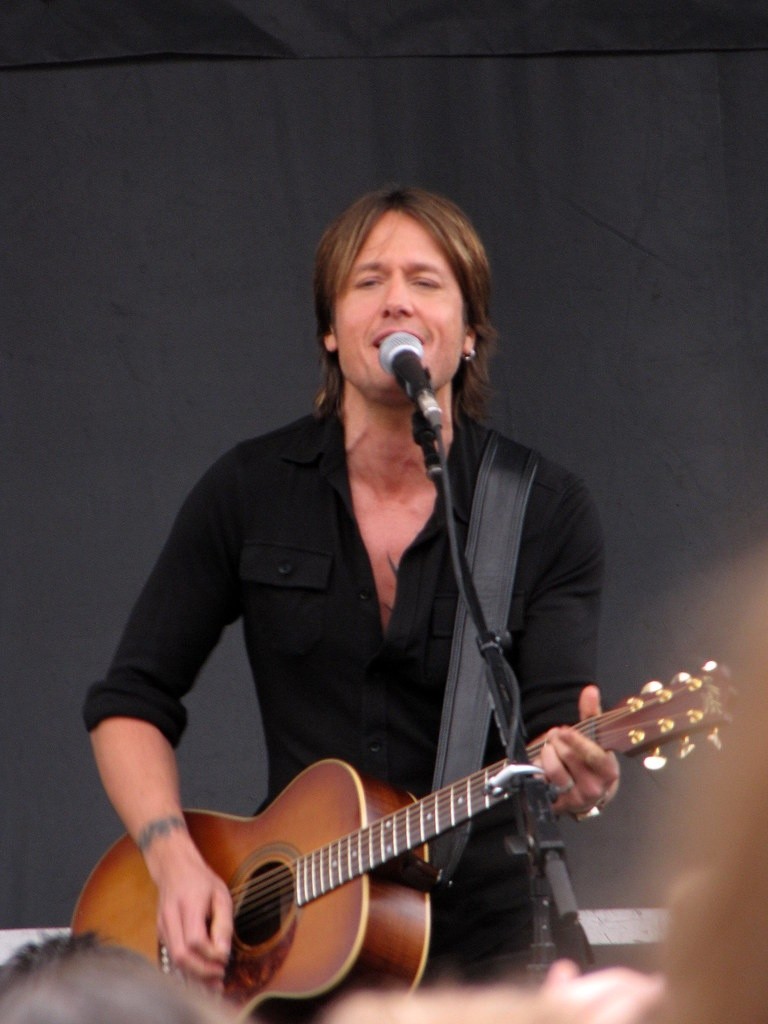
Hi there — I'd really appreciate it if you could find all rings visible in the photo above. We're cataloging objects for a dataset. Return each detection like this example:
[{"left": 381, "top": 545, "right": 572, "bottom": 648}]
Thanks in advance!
[{"left": 550, "top": 780, "right": 575, "bottom": 796}]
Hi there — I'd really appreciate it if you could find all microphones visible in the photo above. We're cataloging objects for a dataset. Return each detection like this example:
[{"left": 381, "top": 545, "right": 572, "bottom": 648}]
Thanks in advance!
[{"left": 379, "top": 331, "right": 443, "bottom": 431}]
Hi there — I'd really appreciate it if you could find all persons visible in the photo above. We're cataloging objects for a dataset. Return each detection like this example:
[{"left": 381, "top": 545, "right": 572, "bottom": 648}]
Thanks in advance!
[{"left": 85, "top": 179, "right": 627, "bottom": 1024}]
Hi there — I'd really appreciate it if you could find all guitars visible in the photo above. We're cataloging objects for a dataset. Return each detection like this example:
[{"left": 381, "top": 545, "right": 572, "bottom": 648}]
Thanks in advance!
[{"left": 69, "top": 655, "right": 745, "bottom": 1023}]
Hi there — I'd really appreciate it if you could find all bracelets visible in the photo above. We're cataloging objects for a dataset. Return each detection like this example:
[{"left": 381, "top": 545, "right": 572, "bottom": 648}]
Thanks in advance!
[{"left": 566, "top": 792, "right": 609, "bottom": 823}]
